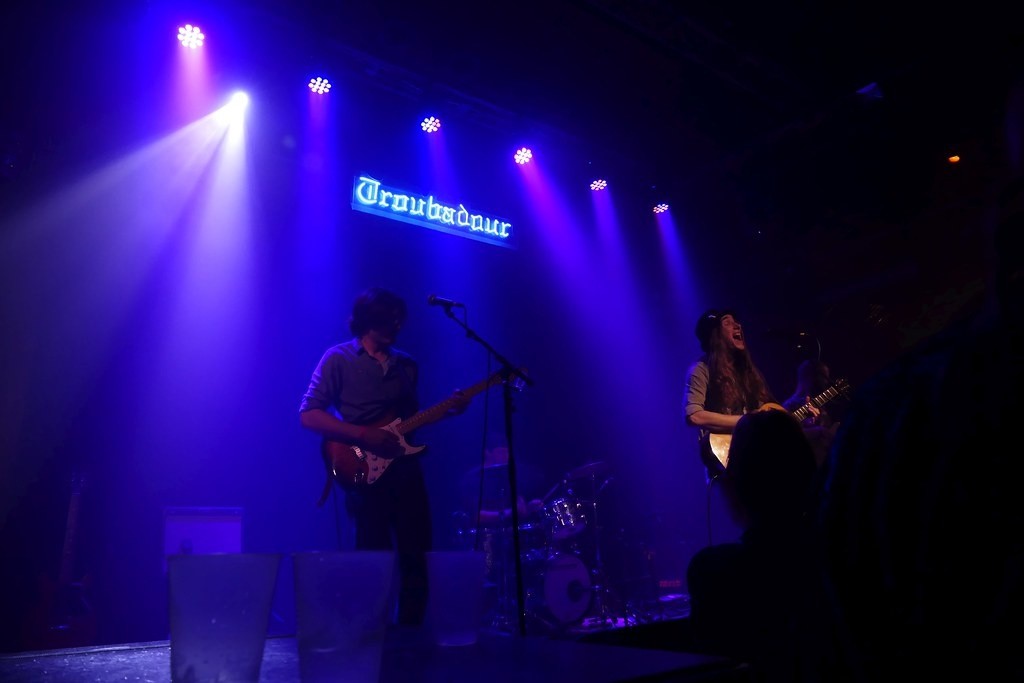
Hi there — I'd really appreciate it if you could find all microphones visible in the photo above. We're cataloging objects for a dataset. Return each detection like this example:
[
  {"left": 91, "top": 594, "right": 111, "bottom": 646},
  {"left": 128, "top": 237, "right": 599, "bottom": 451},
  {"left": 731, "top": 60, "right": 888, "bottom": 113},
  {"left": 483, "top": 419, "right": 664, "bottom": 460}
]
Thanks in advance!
[
  {"left": 765, "top": 328, "right": 808, "bottom": 339},
  {"left": 427, "top": 294, "right": 465, "bottom": 308}
]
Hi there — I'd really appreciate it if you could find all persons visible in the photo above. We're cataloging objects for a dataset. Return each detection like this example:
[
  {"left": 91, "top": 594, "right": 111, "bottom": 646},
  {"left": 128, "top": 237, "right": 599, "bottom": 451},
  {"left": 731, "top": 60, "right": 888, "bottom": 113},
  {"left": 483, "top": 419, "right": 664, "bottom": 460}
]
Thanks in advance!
[
  {"left": 684, "top": 303, "right": 812, "bottom": 478},
  {"left": 783, "top": 358, "right": 841, "bottom": 467},
  {"left": 462, "top": 428, "right": 545, "bottom": 575},
  {"left": 299, "top": 290, "right": 435, "bottom": 630},
  {"left": 687, "top": 411, "right": 885, "bottom": 682}
]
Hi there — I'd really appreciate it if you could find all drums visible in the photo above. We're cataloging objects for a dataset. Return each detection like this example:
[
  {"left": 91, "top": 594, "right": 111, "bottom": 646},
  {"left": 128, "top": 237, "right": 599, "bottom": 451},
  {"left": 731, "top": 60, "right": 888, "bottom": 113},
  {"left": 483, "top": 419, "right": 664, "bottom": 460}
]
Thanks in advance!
[{"left": 454, "top": 484, "right": 604, "bottom": 640}]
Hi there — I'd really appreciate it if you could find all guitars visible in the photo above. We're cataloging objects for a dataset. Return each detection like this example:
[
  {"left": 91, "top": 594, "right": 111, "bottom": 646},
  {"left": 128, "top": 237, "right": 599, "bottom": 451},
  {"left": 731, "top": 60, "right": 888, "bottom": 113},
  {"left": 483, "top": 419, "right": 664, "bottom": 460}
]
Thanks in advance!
[
  {"left": 697, "top": 372, "right": 859, "bottom": 488},
  {"left": 321, "top": 362, "right": 532, "bottom": 496}
]
